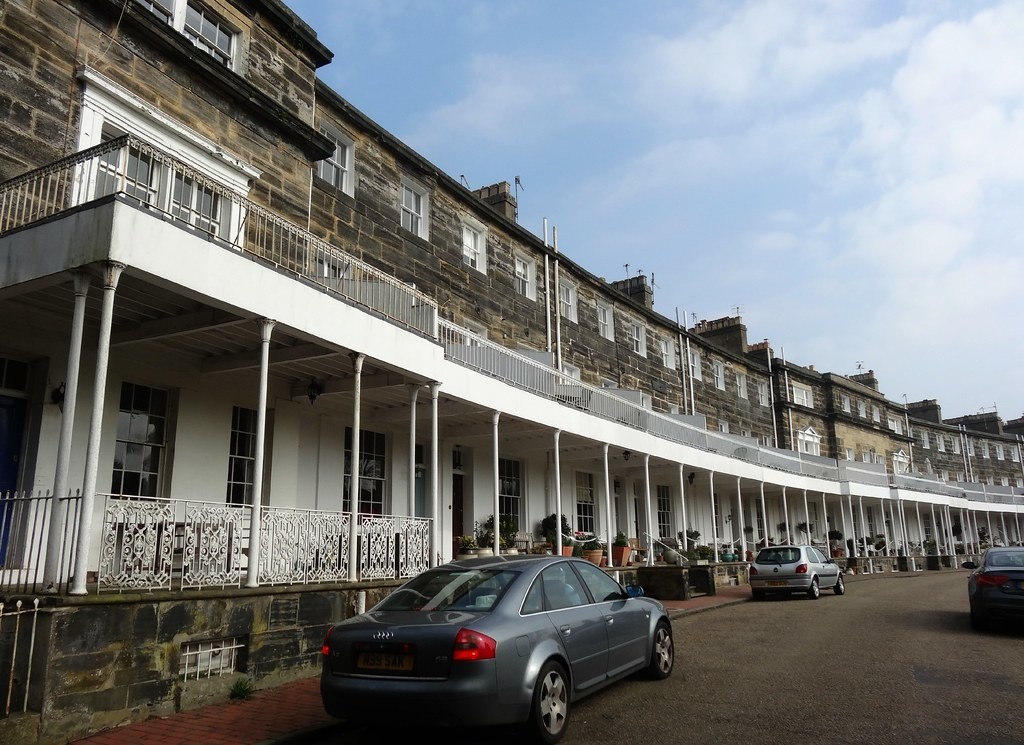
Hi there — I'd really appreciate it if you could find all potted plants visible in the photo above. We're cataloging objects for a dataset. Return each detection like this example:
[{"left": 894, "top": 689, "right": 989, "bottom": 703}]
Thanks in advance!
[{"left": 453, "top": 512, "right": 1024, "bottom": 573}]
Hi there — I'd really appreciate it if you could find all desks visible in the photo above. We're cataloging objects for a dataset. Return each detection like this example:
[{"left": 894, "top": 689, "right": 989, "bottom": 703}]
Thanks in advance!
[{"left": 114, "top": 522, "right": 234, "bottom": 576}]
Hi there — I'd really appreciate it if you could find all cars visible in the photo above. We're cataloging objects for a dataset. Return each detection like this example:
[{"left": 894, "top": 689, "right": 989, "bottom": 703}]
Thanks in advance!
[
  {"left": 748, "top": 544, "right": 845, "bottom": 601},
  {"left": 319, "top": 554, "right": 677, "bottom": 745},
  {"left": 961, "top": 546, "right": 1024, "bottom": 635}
]
